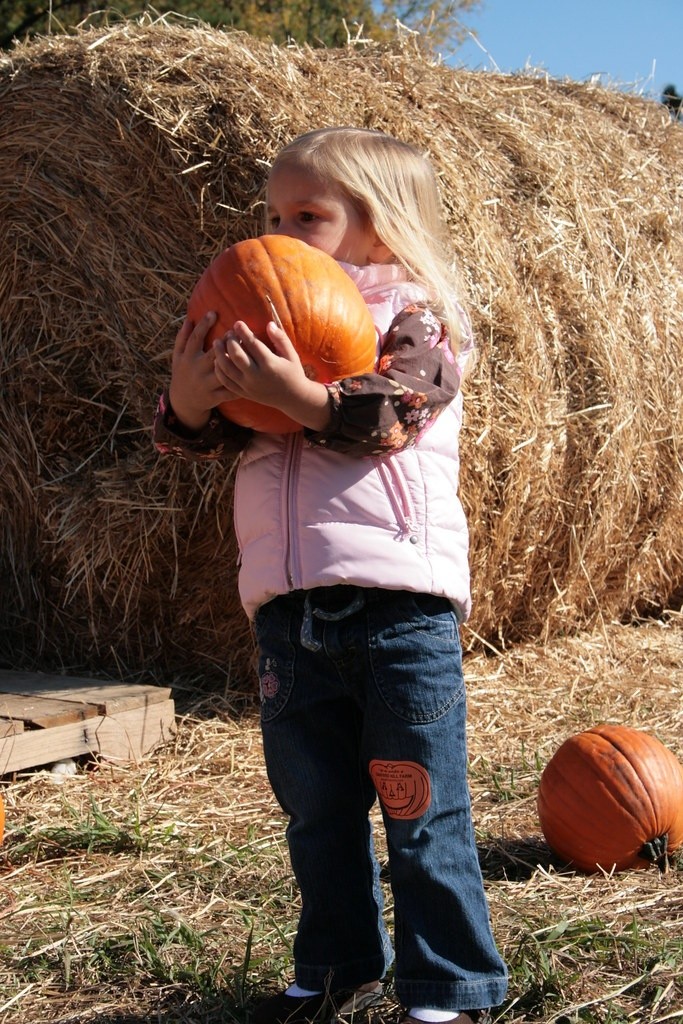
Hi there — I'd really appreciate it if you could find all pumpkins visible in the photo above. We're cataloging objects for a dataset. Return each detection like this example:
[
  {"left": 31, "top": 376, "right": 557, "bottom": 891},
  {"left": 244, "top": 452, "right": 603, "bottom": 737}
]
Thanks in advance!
[
  {"left": 537, "top": 725, "right": 683, "bottom": 875},
  {"left": 186, "top": 234, "right": 375, "bottom": 435}
]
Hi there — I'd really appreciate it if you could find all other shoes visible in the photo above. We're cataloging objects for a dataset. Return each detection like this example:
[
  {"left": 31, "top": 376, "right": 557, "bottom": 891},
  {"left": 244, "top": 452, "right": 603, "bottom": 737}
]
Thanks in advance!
[
  {"left": 252, "top": 979, "right": 379, "bottom": 1024},
  {"left": 399, "top": 1010, "right": 480, "bottom": 1024}
]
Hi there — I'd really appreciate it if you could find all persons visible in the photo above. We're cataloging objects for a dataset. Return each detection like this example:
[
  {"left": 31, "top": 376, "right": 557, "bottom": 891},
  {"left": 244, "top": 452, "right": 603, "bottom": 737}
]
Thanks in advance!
[{"left": 151, "top": 125, "right": 509, "bottom": 1024}]
